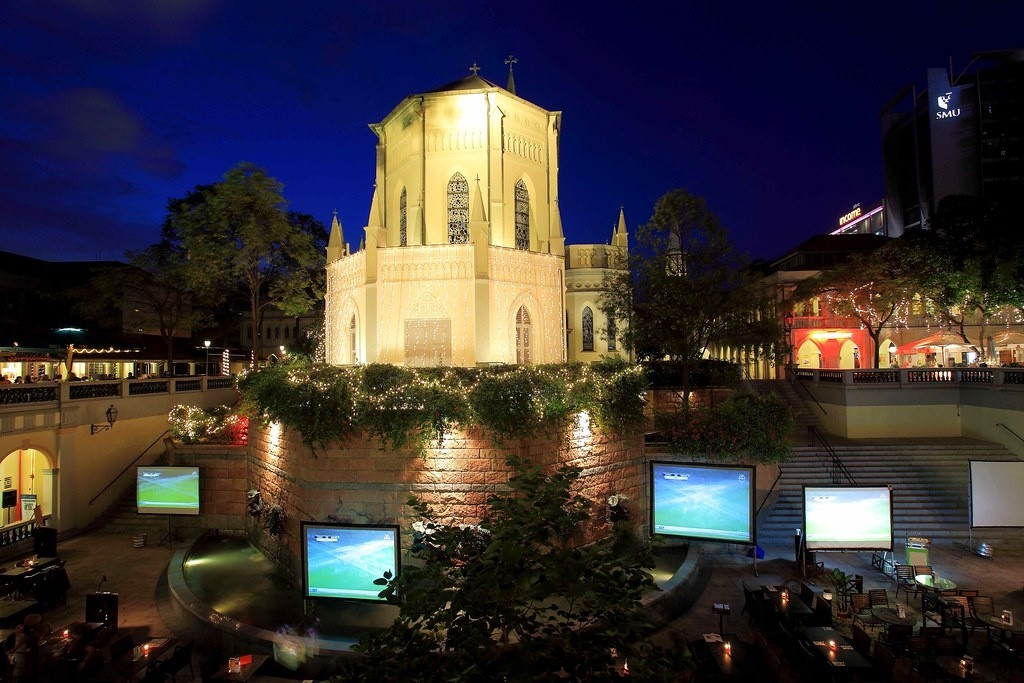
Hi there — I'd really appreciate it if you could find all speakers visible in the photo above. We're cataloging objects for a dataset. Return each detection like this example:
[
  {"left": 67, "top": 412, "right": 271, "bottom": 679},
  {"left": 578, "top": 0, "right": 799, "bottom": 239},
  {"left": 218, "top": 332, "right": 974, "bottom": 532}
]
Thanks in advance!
[
  {"left": 33, "top": 526, "right": 58, "bottom": 556},
  {"left": 2, "top": 489, "right": 18, "bottom": 508}
]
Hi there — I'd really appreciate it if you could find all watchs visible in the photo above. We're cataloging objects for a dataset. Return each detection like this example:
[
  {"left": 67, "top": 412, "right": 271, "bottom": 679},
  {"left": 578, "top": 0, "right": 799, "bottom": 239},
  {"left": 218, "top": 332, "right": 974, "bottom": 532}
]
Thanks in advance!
[{"left": 82, "top": 657, "right": 88, "bottom": 662}]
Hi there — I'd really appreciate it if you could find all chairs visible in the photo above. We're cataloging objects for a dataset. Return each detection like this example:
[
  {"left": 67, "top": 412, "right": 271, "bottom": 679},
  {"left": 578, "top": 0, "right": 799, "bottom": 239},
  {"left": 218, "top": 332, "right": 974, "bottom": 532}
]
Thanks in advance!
[
  {"left": 688, "top": 563, "right": 1024, "bottom": 683},
  {"left": 2, "top": 557, "right": 197, "bottom": 682}
]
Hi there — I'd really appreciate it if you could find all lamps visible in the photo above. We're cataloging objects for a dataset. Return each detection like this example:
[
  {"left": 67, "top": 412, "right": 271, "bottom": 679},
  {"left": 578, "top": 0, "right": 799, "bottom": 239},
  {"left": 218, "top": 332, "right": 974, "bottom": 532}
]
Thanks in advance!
[{"left": 105, "top": 406, "right": 118, "bottom": 426}]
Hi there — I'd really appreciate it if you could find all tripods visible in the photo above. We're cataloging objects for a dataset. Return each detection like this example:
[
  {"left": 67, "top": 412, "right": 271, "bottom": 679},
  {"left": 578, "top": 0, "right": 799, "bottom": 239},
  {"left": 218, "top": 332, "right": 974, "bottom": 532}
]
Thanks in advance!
[{"left": 155, "top": 517, "right": 183, "bottom": 552}]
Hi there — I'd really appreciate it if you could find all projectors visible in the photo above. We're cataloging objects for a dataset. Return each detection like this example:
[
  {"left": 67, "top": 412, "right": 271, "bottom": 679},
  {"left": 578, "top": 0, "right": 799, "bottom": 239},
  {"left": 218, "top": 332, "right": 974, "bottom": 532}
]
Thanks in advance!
[{"left": 712, "top": 602, "right": 730, "bottom": 614}]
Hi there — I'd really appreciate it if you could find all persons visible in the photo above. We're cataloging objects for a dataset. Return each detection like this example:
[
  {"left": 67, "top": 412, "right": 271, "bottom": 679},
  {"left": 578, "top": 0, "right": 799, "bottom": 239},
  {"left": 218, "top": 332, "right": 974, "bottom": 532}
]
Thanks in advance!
[
  {"left": 5, "top": 620, "right": 25, "bottom": 665},
  {"left": 52, "top": 623, "right": 93, "bottom": 673}
]
